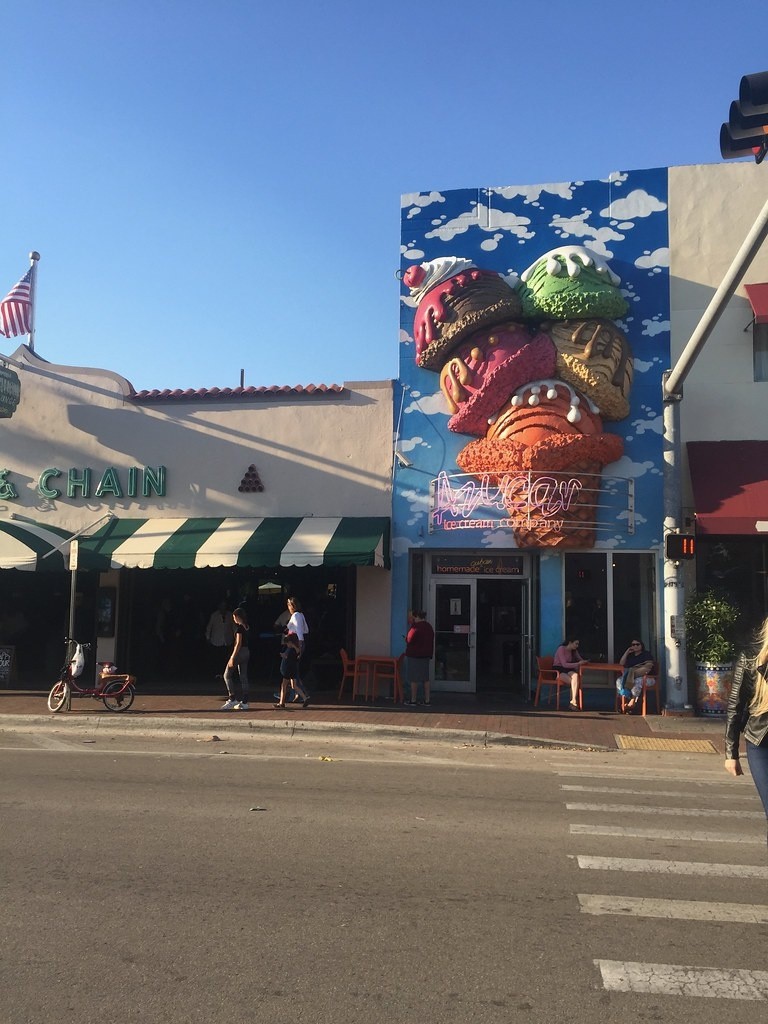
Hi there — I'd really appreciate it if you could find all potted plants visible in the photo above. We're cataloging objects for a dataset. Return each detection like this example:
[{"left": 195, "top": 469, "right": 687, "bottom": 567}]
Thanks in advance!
[{"left": 682, "top": 585, "right": 742, "bottom": 717}]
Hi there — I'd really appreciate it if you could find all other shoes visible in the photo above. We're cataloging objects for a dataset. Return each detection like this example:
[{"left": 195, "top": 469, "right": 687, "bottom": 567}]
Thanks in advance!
[
  {"left": 570, "top": 701, "right": 578, "bottom": 707},
  {"left": 627, "top": 704, "right": 635, "bottom": 710},
  {"left": 272, "top": 703, "right": 287, "bottom": 709},
  {"left": 214, "top": 674, "right": 223, "bottom": 680},
  {"left": 303, "top": 699, "right": 311, "bottom": 707},
  {"left": 418, "top": 700, "right": 431, "bottom": 707},
  {"left": 568, "top": 705, "right": 580, "bottom": 712},
  {"left": 301, "top": 696, "right": 310, "bottom": 702},
  {"left": 622, "top": 704, "right": 632, "bottom": 715},
  {"left": 404, "top": 701, "right": 417, "bottom": 706},
  {"left": 273, "top": 693, "right": 280, "bottom": 699},
  {"left": 290, "top": 699, "right": 298, "bottom": 702}
]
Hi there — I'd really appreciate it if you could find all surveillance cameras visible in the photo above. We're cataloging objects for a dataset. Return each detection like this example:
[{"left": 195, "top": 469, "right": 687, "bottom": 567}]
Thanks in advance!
[{"left": 396, "top": 450, "right": 413, "bottom": 466}]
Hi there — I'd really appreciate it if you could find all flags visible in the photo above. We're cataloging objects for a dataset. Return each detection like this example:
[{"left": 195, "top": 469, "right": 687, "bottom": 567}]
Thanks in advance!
[{"left": 1, "top": 264, "right": 35, "bottom": 338}]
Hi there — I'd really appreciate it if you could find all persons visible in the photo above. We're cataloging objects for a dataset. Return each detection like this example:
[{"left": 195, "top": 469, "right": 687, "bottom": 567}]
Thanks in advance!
[
  {"left": 615, "top": 638, "right": 656, "bottom": 715},
  {"left": 725, "top": 615, "right": 768, "bottom": 820},
  {"left": 221, "top": 600, "right": 253, "bottom": 703},
  {"left": 401, "top": 609, "right": 434, "bottom": 707},
  {"left": 274, "top": 596, "right": 312, "bottom": 708},
  {"left": 565, "top": 599, "right": 579, "bottom": 640},
  {"left": 206, "top": 600, "right": 236, "bottom": 680},
  {"left": 552, "top": 633, "right": 589, "bottom": 711}
]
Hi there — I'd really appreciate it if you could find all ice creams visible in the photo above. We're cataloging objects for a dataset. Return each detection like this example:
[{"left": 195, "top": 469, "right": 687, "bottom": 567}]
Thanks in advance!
[{"left": 394, "top": 245, "right": 634, "bottom": 548}]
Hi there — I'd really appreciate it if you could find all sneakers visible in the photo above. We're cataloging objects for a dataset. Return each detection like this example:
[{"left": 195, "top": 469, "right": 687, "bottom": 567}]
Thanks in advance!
[
  {"left": 234, "top": 701, "right": 248, "bottom": 710},
  {"left": 221, "top": 699, "right": 238, "bottom": 709}
]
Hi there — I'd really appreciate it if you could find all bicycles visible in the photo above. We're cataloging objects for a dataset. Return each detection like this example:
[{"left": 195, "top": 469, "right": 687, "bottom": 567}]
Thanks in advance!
[{"left": 47, "top": 635, "right": 137, "bottom": 713}]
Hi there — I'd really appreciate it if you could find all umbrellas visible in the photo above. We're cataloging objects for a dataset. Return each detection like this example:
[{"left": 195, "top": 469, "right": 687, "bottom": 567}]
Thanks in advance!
[{"left": 0, "top": 516, "right": 79, "bottom": 573}]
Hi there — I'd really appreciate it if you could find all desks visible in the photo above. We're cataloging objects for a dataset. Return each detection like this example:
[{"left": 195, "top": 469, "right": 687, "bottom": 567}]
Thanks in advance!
[
  {"left": 352, "top": 656, "right": 397, "bottom": 703},
  {"left": 579, "top": 663, "right": 625, "bottom": 713}
]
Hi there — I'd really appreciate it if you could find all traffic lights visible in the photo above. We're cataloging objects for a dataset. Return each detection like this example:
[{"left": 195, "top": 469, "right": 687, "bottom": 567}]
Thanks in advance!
[
  {"left": 720, "top": 70, "right": 768, "bottom": 164},
  {"left": 664, "top": 533, "right": 697, "bottom": 560}
]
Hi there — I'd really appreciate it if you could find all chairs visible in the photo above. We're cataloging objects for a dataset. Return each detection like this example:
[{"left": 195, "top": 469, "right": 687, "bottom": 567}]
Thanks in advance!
[
  {"left": 534, "top": 655, "right": 570, "bottom": 711},
  {"left": 338, "top": 648, "right": 369, "bottom": 701},
  {"left": 615, "top": 663, "right": 661, "bottom": 716},
  {"left": 372, "top": 651, "right": 405, "bottom": 702}
]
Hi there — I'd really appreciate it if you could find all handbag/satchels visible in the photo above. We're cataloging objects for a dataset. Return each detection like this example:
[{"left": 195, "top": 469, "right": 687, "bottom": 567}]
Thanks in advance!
[{"left": 69, "top": 644, "right": 85, "bottom": 678}]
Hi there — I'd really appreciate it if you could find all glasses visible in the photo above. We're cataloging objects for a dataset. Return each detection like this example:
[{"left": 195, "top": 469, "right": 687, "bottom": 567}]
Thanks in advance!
[{"left": 632, "top": 643, "right": 640, "bottom": 647}]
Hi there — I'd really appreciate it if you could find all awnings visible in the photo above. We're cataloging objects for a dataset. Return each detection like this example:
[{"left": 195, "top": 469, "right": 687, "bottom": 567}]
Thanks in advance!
[
  {"left": 686, "top": 440, "right": 768, "bottom": 535},
  {"left": 76, "top": 516, "right": 391, "bottom": 572}
]
